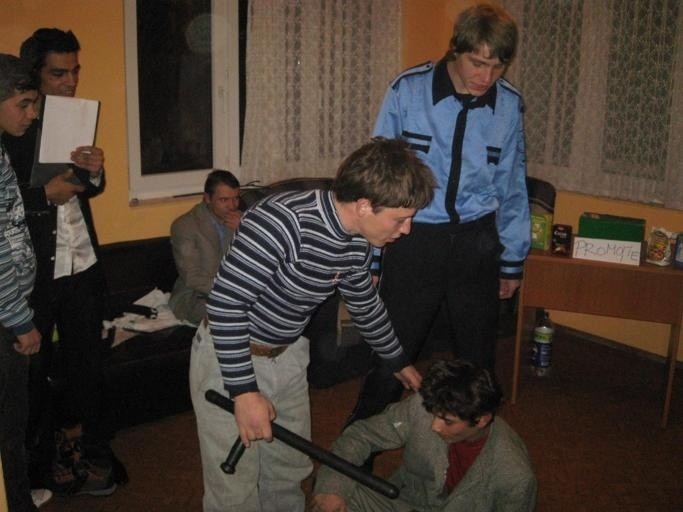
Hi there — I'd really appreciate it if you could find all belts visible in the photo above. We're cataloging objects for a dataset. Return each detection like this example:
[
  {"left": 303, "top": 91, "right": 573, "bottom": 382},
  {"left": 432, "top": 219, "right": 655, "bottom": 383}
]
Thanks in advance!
[{"left": 242, "top": 342, "right": 288, "bottom": 358}]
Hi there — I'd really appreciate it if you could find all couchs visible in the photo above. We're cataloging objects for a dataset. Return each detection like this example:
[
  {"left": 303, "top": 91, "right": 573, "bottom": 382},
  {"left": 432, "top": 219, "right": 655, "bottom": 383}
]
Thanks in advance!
[{"left": 34, "top": 236, "right": 310, "bottom": 447}]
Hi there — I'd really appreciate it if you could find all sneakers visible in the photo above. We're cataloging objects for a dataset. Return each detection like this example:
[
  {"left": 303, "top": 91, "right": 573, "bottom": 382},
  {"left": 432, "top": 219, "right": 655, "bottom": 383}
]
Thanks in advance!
[{"left": 27, "top": 426, "right": 118, "bottom": 497}]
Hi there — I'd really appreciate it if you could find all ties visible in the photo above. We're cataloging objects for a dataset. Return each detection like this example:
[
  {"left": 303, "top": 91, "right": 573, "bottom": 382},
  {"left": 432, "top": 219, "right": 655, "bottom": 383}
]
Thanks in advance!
[{"left": 444, "top": 97, "right": 486, "bottom": 223}]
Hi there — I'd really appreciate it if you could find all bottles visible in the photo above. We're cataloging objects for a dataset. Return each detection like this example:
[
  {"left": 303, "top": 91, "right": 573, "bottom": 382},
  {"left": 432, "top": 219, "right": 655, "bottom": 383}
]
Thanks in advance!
[{"left": 532, "top": 311, "right": 554, "bottom": 375}]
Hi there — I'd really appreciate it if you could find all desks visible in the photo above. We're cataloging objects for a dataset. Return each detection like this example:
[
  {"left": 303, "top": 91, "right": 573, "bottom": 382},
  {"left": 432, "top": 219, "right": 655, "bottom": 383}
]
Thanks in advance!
[
  {"left": 541, "top": 308, "right": 683, "bottom": 367},
  {"left": 509, "top": 250, "right": 682, "bottom": 427}
]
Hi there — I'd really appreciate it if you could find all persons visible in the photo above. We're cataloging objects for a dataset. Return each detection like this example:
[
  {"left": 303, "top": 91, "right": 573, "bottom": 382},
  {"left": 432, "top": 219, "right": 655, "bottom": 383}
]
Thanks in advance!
[
  {"left": 311, "top": 358, "right": 539, "bottom": 512},
  {"left": 189, "top": 134, "right": 436, "bottom": 512},
  {"left": 0, "top": 26, "right": 106, "bottom": 497},
  {"left": 169, "top": 169, "right": 245, "bottom": 326},
  {"left": 342, "top": 5, "right": 531, "bottom": 478},
  {"left": 0, "top": 52, "right": 42, "bottom": 512}
]
